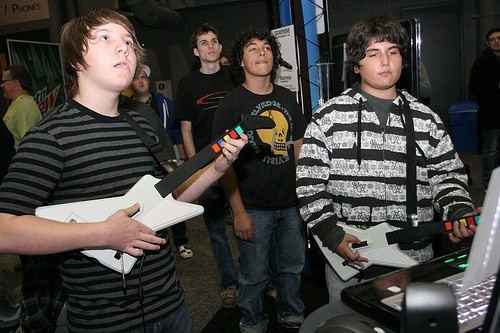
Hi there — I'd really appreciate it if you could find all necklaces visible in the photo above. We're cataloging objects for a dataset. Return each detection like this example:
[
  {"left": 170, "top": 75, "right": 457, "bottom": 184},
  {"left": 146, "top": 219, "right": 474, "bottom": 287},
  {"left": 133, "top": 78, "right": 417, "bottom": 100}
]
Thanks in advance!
[{"left": 133, "top": 94, "right": 152, "bottom": 107}]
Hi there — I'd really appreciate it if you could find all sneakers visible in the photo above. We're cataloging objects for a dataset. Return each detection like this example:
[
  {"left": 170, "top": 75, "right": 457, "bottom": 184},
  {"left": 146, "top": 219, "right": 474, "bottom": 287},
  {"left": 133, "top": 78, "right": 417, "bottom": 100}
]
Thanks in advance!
[
  {"left": 220, "top": 285, "right": 239, "bottom": 308},
  {"left": 178, "top": 244, "right": 193, "bottom": 259}
]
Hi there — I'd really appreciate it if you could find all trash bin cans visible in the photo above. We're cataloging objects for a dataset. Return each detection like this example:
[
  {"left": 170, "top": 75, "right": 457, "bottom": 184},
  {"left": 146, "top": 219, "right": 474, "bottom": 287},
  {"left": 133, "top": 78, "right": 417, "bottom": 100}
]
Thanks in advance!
[{"left": 449, "top": 104, "right": 479, "bottom": 154}]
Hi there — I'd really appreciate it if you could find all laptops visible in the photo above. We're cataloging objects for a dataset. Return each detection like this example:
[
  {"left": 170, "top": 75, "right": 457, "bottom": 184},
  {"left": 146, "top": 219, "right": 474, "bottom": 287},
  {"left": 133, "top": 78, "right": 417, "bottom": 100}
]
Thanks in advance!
[{"left": 341, "top": 166, "right": 500, "bottom": 333}]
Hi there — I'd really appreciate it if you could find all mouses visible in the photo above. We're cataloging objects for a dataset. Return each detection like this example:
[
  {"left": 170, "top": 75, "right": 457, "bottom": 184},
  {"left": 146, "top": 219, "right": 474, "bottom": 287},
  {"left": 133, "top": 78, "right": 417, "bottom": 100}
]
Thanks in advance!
[{"left": 315, "top": 312, "right": 391, "bottom": 333}]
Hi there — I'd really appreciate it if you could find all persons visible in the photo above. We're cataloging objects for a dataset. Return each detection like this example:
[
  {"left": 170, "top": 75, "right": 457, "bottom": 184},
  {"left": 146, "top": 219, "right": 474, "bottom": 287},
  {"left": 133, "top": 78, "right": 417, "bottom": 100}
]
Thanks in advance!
[
  {"left": 467, "top": 28, "right": 500, "bottom": 192},
  {"left": 179, "top": 24, "right": 278, "bottom": 308},
  {"left": 220, "top": 53, "right": 233, "bottom": 226},
  {"left": 211, "top": 29, "right": 308, "bottom": 333},
  {"left": 295, "top": 18, "right": 483, "bottom": 305},
  {"left": 0, "top": 8, "right": 250, "bottom": 333},
  {"left": 128, "top": 60, "right": 194, "bottom": 261},
  {"left": 0, "top": 66, "right": 46, "bottom": 333}
]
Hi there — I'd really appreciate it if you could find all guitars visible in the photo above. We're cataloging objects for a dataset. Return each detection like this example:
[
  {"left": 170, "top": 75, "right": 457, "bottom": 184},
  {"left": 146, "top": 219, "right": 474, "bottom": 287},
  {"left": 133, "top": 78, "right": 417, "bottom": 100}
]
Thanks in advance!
[
  {"left": 313, "top": 215, "right": 481, "bottom": 282},
  {"left": 35, "top": 114, "right": 276, "bottom": 274}
]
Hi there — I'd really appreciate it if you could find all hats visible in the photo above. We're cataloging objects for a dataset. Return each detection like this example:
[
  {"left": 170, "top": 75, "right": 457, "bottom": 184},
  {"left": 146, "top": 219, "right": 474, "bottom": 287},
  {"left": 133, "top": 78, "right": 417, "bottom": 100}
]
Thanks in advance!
[{"left": 141, "top": 64, "right": 150, "bottom": 77}]
